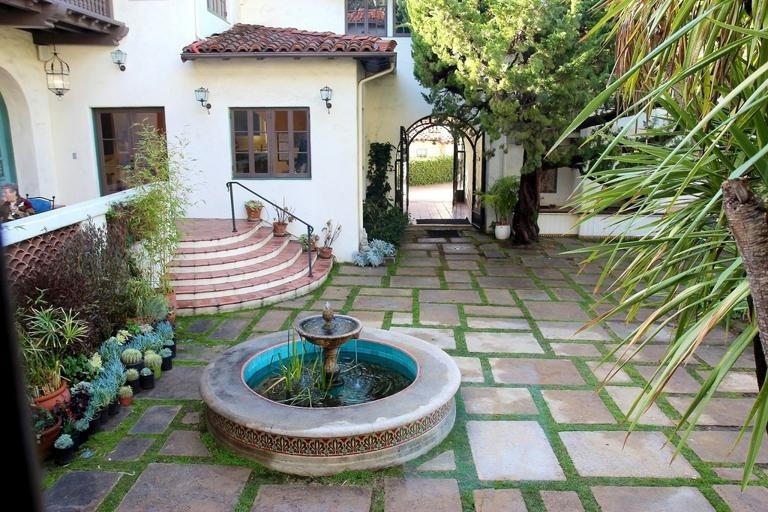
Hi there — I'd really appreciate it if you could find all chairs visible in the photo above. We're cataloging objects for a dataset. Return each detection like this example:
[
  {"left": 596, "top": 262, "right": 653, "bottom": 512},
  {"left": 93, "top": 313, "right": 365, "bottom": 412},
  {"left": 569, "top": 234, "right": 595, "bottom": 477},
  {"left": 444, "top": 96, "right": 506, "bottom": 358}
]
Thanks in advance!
[{"left": 26, "top": 194, "right": 55, "bottom": 214}]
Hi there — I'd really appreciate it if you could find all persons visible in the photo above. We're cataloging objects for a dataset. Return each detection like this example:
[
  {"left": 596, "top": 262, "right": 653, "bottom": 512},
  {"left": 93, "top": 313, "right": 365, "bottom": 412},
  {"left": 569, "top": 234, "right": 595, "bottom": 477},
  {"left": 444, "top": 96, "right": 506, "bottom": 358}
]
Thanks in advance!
[{"left": 0, "top": 184, "right": 35, "bottom": 221}]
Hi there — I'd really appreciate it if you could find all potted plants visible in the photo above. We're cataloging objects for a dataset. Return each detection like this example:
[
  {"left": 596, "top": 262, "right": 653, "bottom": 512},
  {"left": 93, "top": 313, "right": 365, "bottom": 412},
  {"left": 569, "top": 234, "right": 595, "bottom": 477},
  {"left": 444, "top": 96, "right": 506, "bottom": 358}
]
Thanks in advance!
[
  {"left": 12, "top": 305, "right": 177, "bottom": 467},
  {"left": 299, "top": 234, "right": 319, "bottom": 252},
  {"left": 273, "top": 196, "right": 296, "bottom": 237},
  {"left": 473, "top": 175, "right": 522, "bottom": 240},
  {"left": 244, "top": 200, "right": 265, "bottom": 221},
  {"left": 320, "top": 219, "right": 343, "bottom": 258}
]
